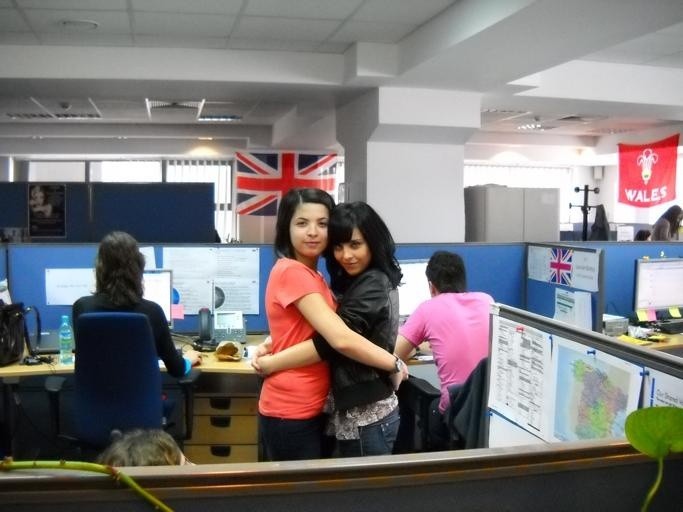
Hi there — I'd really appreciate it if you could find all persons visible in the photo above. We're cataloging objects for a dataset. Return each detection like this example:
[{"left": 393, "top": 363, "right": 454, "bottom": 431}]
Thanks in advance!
[
  {"left": 650, "top": 205, "right": 683, "bottom": 241},
  {"left": 69, "top": 230, "right": 203, "bottom": 429},
  {"left": 633, "top": 229, "right": 651, "bottom": 242},
  {"left": 96, "top": 428, "right": 197, "bottom": 468},
  {"left": 394, "top": 250, "right": 495, "bottom": 453}
]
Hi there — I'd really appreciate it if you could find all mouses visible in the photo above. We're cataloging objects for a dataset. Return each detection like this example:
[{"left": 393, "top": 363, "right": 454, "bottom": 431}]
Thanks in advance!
[{"left": 192, "top": 357, "right": 202, "bottom": 366}]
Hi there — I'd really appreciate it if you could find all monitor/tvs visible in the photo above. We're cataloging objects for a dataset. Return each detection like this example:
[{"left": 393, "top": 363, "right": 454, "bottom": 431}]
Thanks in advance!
[
  {"left": 396, "top": 258, "right": 432, "bottom": 324},
  {"left": 632, "top": 257, "right": 683, "bottom": 322},
  {"left": 141, "top": 269, "right": 173, "bottom": 328}
]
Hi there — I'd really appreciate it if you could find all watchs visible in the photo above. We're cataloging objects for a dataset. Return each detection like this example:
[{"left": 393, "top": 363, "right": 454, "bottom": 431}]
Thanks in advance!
[{"left": 387, "top": 354, "right": 402, "bottom": 377}]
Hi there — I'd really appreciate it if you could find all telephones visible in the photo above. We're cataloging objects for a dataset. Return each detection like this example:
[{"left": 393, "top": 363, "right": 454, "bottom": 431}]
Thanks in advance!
[{"left": 194, "top": 308, "right": 246, "bottom": 346}]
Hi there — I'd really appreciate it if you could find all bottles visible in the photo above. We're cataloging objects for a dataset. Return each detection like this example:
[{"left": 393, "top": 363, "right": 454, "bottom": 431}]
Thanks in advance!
[{"left": 59, "top": 315, "right": 73, "bottom": 367}]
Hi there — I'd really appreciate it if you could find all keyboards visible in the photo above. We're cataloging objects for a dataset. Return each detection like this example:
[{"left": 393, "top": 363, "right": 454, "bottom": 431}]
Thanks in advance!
[
  {"left": 656, "top": 321, "right": 683, "bottom": 334},
  {"left": 175, "top": 346, "right": 183, "bottom": 359}
]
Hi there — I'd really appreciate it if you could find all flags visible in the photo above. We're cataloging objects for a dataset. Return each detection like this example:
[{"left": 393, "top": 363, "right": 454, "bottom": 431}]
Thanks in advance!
[
  {"left": 549, "top": 247, "right": 573, "bottom": 286},
  {"left": 233, "top": 150, "right": 337, "bottom": 216}
]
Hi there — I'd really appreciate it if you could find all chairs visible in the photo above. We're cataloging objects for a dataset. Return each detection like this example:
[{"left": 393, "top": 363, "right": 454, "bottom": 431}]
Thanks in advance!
[
  {"left": 402, "top": 357, "right": 488, "bottom": 452},
  {"left": 46, "top": 312, "right": 201, "bottom": 457}
]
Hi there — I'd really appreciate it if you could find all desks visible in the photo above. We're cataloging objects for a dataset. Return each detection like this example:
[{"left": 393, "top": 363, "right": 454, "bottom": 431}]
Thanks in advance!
[
  {"left": 407, "top": 340, "right": 434, "bottom": 364},
  {"left": 600, "top": 316, "right": 682, "bottom": 352},
  {"left": 0, "top": 330, "right": 270, "bottom": 393}
]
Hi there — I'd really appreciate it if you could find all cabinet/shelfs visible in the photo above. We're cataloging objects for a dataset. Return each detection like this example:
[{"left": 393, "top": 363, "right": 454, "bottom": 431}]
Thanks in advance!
[{"left": 180, "top": 392, "right": 262, "bottom": 465}]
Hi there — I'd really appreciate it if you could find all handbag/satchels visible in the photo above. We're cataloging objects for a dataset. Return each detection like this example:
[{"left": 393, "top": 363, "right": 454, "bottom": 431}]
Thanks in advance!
[{"left": 0, "top": 298, "right": 41, "bottom": 367}]
